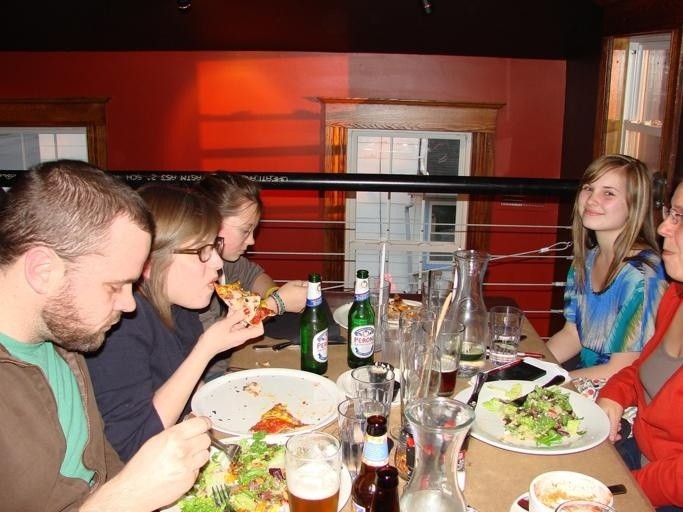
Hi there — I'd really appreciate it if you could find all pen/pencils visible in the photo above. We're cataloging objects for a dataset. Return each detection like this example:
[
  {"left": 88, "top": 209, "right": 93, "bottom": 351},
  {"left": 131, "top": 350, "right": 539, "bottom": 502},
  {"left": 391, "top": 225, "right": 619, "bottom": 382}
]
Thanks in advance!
[
  {"left": 488, "top": 359, "right": 523, "bottom": 376},
  {"left": 486, "top": 349, "right": 543, "bottom": 358}
]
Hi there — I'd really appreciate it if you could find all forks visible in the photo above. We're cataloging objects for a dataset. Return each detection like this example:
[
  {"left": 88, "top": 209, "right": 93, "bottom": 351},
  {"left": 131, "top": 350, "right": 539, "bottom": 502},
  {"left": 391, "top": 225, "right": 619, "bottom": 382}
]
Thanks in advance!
[
  {"left": 182, "top": 413, "right": 242, "bottom": 467},
  {"left": 497, "top": 374, "right": 566, "bottom": 408},
  {"left": 211, "top": 483, "right": 234, "bottom": 512},
  {"left": 272, "top": 334, "right": 347, "bottom": 353}
]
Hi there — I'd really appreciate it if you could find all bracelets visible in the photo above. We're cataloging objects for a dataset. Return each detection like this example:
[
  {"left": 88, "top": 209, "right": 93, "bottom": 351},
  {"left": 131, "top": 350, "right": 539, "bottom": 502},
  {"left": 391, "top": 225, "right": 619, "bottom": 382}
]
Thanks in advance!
[{"left": 268, "top": 292, "right": 287, "bottom": 316}]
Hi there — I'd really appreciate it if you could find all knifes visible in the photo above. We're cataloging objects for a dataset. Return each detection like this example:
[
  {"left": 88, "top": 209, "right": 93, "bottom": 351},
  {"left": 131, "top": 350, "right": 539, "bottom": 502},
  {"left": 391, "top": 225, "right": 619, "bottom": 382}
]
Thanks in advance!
[{"left": 253, "top": 341, "right": 346, "bottom": 352}]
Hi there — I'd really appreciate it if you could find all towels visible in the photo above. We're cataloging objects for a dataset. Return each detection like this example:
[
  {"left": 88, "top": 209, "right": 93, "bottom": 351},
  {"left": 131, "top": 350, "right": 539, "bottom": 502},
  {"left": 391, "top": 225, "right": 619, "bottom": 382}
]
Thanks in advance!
[{"left": 469, "top": 356, "right": 569, "bottom": 387}]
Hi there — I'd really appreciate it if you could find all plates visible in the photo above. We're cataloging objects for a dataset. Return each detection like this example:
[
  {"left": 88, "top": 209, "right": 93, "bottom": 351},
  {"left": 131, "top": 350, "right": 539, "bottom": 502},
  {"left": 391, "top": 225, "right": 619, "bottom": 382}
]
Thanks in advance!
[
  {"left": 190, "top": 367, "right": 349, "bottom": 435},
  {"left": 159, "top": 435, "right": 353, "bottom": 512},
  {"left": 334, "top": 365, "right": 419, "bottom": 409},
  {"left": 452, "top": 381, "right": 611, "bottom": 455},
  {"left": 509, "top": 492, "right": 531, "bottom": 512},
  {"left": 332, "top": 298, "right": 423, "bottom": 331}
]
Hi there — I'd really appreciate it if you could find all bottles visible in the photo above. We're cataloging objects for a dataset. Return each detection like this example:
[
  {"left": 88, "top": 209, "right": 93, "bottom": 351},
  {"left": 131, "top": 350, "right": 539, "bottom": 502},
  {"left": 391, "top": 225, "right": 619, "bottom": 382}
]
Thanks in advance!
[
  {"left": 348, "top": 270, "right": 375, "bottom": 370},
  {"left": 300, "top": 272, "right": 328, "bottom": 375},
  {"left": 351, "top": 415, "right": 391, "bottom": 511},
  {"left": 369, "top": 466, "right": 400, "bottom": 512}
]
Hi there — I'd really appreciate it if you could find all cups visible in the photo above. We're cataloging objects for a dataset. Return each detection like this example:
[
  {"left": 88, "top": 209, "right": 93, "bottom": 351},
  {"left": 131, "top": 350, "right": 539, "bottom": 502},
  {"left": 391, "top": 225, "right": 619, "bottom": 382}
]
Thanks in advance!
[
  {"left": 397, "top": 309, "right": 434, "bottom": 369},
  {"left": 529, "top": 471, "right": 613, "bottom": 511},
  {"left": 354, "top": 277, "right": 391, "bottom": 355},
  {"left": 337, "top": 397, "right": 388, "bottom": 482},
  {"left": 349, "top": 365, "right": 396, "bottom": 434},
  {"left": 555, "top": 499, "right": 617, "bottom": 512},
  {"left": 421, "top": 278, "right": 454, "bottom": 330},
  {"left": 490, "top": 305, "right": 524, "bottom": 365},
  {"left": 430, "top": 317, "right": 466, "bottom": 399},
  {"left": 284, "top": 432, "right": 343, "bottom": 511}
]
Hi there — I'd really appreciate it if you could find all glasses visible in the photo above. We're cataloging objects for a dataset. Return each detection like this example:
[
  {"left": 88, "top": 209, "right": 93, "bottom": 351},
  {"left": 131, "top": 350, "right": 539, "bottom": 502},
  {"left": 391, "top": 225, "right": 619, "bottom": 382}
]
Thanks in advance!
[
  {"left": 163, "top": 238, "right": 226, "bottom": 262},
  {"left": 660, "top": 205, "right": 683, "bottom": 228}
]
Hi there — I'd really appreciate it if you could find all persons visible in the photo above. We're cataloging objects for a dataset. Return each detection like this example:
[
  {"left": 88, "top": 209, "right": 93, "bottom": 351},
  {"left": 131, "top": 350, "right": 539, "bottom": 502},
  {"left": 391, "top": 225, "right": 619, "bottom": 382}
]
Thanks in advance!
[
  {"left": 544, "top": 155, "right": 665, "bottom": 391},
  {"left": 85, "top": 180, "right": 264, "bottom": 466},
  {"left": 0, "top": 158, "right": 212, "bottom": 512},
  {"left": 193, "top": 170, "right": 309, "bottom": 382},
  {"left": 596, "top": 173, "right": 683, "bottom": 511}
]
